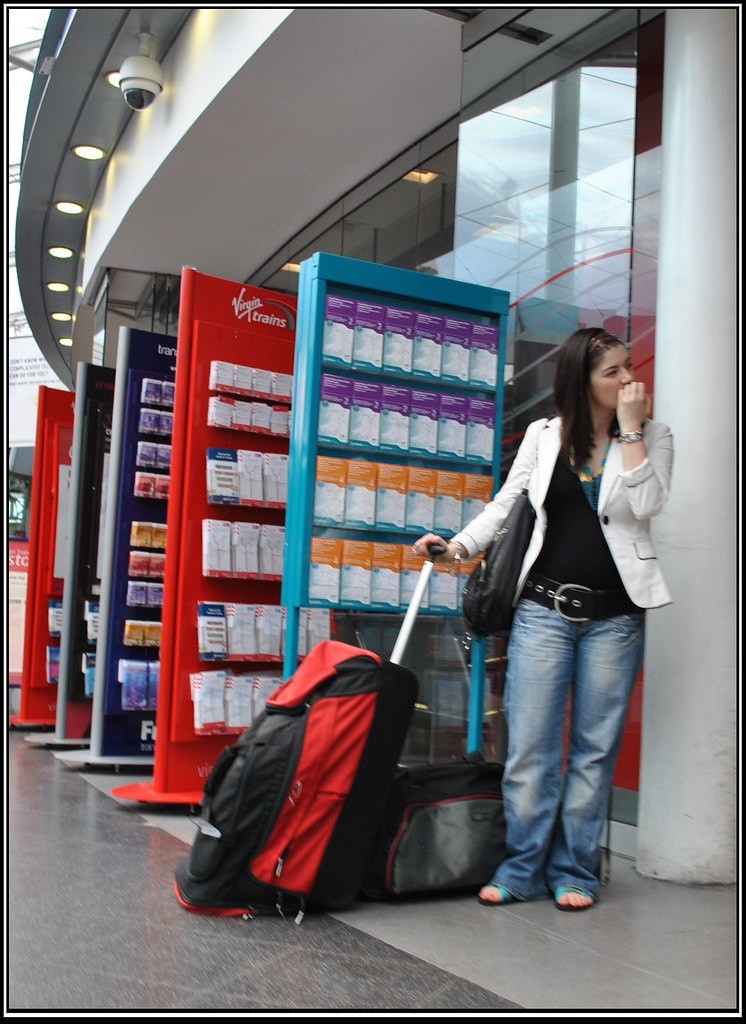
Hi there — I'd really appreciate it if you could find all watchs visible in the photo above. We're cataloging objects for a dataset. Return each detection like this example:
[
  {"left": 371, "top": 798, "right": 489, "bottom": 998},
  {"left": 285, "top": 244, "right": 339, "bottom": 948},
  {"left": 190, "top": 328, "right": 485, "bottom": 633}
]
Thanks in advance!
[{"left": 619, "top": 431, "right": 643, "bottom": 441}]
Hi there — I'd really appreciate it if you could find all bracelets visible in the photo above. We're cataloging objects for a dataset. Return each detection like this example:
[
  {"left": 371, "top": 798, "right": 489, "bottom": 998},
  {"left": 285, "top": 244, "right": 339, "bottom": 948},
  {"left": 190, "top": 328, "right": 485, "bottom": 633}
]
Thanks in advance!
[{"left": 445, "top": 541, "right": 462, "bottom": 576}]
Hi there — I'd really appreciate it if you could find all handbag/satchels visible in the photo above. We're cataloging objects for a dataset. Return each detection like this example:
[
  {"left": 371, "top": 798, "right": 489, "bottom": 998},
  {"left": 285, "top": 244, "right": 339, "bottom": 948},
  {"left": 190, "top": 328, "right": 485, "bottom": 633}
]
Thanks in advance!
[
  {"left": 461, "top": 488, "right": 537, "bottom": 638},
  {"left": 358, "top": 751, "right": 506, "bottom": 903}
]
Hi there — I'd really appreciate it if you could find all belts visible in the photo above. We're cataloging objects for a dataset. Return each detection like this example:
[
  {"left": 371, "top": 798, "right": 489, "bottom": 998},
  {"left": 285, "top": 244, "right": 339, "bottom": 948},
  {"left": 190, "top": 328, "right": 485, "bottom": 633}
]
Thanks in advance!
[{"left": 520, "top": 573, "right": 646, "bottom": 623}]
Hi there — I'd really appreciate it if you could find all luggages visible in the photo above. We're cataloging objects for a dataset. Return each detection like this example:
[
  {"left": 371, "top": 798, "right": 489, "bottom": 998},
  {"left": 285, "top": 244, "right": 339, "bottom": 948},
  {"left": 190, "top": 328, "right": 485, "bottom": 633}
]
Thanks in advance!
[{"left": 173, "top": 544, "right": 448, "bottom": 926}]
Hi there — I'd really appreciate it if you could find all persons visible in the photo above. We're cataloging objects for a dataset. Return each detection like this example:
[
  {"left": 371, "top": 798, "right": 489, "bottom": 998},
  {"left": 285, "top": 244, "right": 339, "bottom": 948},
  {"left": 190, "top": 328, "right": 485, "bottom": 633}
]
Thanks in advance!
[{"left": 412, "top": 328, "right": 674, "bottom": 911}]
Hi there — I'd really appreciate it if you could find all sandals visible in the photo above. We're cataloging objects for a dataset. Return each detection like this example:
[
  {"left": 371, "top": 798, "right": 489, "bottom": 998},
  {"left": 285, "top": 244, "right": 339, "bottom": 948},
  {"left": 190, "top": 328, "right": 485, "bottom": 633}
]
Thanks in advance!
[
  {"left": 476, "top": 882, "right": 514, "bottom": 906},
  {"left": 553, "top": 886, "right": 596, "bottom": 911}
]
